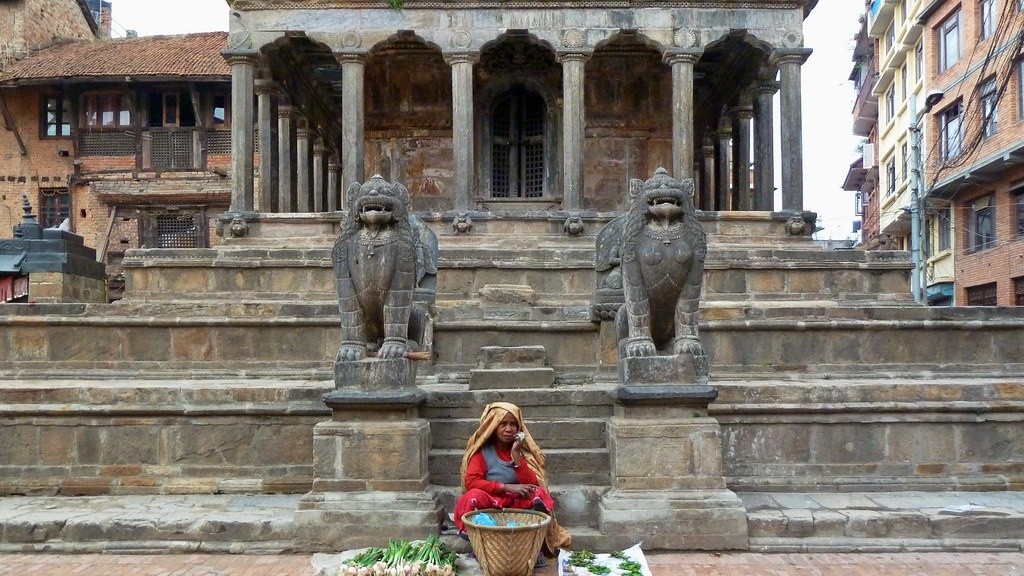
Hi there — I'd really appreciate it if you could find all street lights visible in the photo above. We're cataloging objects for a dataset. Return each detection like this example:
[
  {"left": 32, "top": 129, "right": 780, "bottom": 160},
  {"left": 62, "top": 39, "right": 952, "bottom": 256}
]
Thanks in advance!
[{"left": 907, "top": 88, "right": 943, "bottom": 303}]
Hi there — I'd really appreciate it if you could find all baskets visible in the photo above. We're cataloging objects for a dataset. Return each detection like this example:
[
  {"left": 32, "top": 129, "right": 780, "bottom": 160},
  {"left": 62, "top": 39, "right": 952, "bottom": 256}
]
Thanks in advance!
[{"left": 461, "top": 507, "right": 552, "bottom": 576}]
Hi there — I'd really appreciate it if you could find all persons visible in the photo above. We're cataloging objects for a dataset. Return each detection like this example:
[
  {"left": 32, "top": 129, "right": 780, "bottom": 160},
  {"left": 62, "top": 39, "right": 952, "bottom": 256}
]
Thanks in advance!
[{"left": 452, "top": 401, "right": 556, "bottom": 568}]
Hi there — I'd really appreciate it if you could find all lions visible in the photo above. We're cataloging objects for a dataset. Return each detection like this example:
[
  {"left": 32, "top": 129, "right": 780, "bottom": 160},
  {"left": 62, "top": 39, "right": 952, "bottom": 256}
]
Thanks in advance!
[
  {"left": 593, "top": 166, "right": 708, "bottom": 356},
  {"left": 332, "top": 172, "right": 438, "bottom": 358}
]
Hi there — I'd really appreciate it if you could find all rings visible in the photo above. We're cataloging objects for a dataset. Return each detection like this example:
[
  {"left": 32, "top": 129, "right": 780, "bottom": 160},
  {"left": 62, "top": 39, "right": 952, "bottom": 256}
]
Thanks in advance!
[{"left": 518, "top": 432, "right": 526, "bottom": 442}]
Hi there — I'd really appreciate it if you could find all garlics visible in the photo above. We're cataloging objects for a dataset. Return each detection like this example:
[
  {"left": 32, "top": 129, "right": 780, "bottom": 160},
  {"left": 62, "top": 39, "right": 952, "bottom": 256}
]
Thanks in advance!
[{"left": 337, "top": 559, "right": 456, "bottom": 576}]
